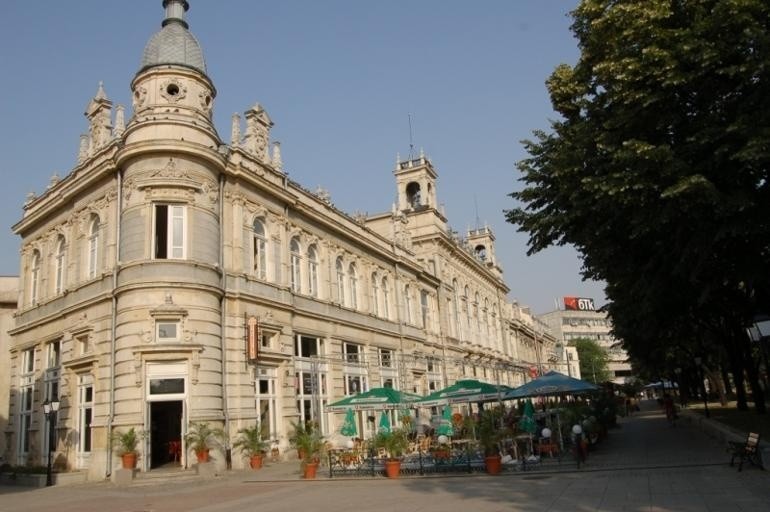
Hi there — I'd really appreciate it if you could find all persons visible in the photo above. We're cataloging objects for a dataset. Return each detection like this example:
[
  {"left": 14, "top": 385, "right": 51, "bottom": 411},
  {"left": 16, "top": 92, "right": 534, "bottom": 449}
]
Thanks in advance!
[{"left": 662, "top": 393, "right": 679, "bottom": 423}]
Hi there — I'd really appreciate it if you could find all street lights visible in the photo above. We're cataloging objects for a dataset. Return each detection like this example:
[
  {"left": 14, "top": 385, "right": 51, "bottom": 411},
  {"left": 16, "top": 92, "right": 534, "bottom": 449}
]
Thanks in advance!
[
  {"left": 673, "top": 364, "right": 683, "bottom": 402},
  {"left": 693, "top": 341, "right": 712, "bottom": 417},
  {"left": 592, "top": 357, "right": 599, "bottom": 384},
  {"left": 41, "top": 394, "right": 62, "bottom": 486},
  {"left": 744, "top": 306, "right": 770, "bottom": 391},
  {"left": 532, "top": 322, "right": 578, "bottom": 405}
]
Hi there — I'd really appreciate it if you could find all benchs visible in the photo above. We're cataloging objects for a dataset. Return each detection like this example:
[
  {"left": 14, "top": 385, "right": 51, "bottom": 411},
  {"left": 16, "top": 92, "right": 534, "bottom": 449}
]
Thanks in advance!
[{"left": 725, "top": 431, "right": 765, "bottom": 472}]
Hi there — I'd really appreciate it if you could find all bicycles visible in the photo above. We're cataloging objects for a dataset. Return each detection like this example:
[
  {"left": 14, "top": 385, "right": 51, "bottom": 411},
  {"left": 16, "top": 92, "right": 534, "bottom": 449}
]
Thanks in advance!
[{"left": 664, "top": 404, "right": 677, "bottom": 428}]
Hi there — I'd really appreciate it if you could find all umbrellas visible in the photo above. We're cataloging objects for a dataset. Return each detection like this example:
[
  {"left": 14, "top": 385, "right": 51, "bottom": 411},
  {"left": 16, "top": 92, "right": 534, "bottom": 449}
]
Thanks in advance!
[{"left": 325, "top": 370, "right": 605, "bottom": 456}]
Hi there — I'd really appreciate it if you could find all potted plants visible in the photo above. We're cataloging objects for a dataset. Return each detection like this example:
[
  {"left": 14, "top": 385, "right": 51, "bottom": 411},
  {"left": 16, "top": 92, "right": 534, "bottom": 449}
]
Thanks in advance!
[
  {"left": 117, "top": 428, "right": 141, "bottom": 470},
  {"left": 482, "top": 431, "right": 502, "bottom": 474},
  {"left": 375, "top": 430, "right": 410, "bottom": 478},
  {"left": 185, "top": 422, "right": 267, "bottom": 470},
  {"left": 289, "top": 420, "right": 329, "bottom": 478}
]
